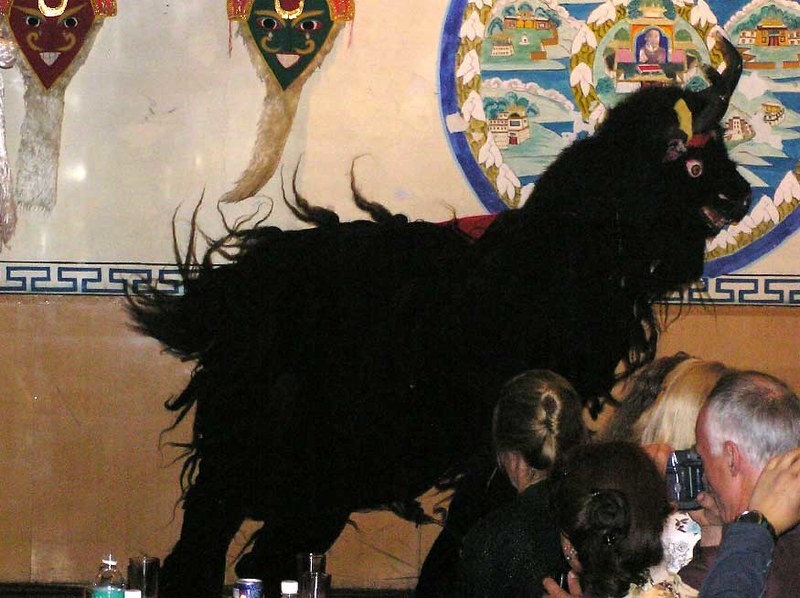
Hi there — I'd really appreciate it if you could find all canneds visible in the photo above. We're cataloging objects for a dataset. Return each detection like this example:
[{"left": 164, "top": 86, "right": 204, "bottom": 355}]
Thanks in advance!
[{"left": 232, "top": 579, "right": 265, "bottom": 598}]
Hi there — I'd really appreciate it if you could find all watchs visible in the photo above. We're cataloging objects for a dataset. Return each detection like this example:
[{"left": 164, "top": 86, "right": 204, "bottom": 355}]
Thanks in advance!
[{"left": 735, "top": 510, "right": 778, "bottom": 545}]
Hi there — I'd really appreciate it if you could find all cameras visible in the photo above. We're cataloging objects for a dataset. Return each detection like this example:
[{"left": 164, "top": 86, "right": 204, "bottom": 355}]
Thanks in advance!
[{"left": 665, "top": 449, "right": 711, "bottom": 511}]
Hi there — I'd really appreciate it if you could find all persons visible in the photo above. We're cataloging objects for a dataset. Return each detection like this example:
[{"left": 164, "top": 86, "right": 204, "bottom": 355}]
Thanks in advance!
[{"left": 453, "top": 350, "right": 800, "bottom": 597}]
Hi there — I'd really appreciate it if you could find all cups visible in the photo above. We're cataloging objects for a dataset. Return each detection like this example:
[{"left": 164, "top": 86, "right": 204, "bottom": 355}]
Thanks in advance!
[
  {"left": 297, "top": 553, "right": 325, "bottom": 572},
  {"left": 129, "top": 556, "right": 160, "bottom": 598},
  {"left": 299, "top": 572, "right": 330, "bottom": 598}
]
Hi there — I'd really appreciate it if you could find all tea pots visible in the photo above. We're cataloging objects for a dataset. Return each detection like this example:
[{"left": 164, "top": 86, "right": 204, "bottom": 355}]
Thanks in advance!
[{"left": 90, "top": 556, "right": 125, "bottom": 598}]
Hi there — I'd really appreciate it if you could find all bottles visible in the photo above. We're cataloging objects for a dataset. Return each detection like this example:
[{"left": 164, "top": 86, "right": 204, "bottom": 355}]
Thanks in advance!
[
  {"left": 281, "top": 580, "right": 299, "bottom": 598},
  {"left": 124, "top": 589, "right": 141, "bottom": 598}
]
